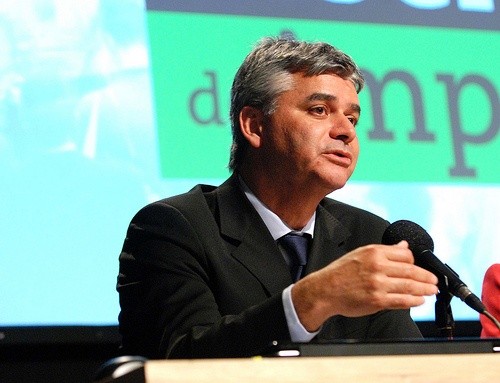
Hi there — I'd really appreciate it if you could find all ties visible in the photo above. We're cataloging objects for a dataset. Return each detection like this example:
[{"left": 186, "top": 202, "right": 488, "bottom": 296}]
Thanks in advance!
[{"left": 278, "top": 231, "right": 313, "bottom": 283}]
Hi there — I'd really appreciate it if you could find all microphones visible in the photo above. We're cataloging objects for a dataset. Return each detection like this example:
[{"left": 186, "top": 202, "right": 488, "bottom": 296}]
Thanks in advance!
[{"left": 381, "top": 220, "right": 487, "bottom": 313}]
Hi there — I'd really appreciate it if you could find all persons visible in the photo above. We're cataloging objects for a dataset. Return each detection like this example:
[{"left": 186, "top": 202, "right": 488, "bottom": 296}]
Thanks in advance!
[{"left": 116, "top": 36, "right": 439, "bottom": 359}]
